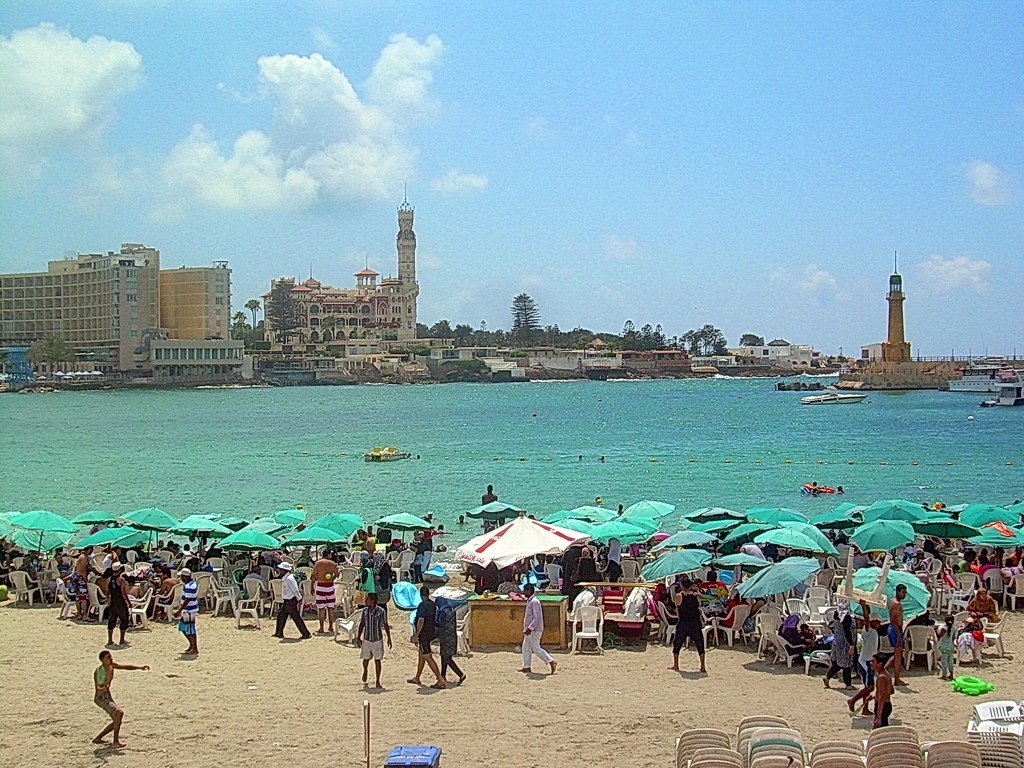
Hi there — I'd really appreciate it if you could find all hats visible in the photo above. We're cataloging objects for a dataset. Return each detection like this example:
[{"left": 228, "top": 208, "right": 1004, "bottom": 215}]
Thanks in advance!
[
  {"left": 906, "top": 542, "right": 917, "bottom": 547},
  {"left": 177, "top": 568, "right": 192, "bottom": 576},
  {"left": 916, "top": 548, "right": 924, "bottom": 553},
  {"left": 278, "top": 561, "right": 292, "bottom": 570},
  {"left": 112, "top": 562, "right": 123, "bottom": 571},
  {"left": 137, "top": 541, "right": 146, "bottom": 547},
  {"left": 360, "top": 550, "right": 369, "bottom": 558},
  {"left": 870, "top": 613, "right": 888, "bottom": 621}
]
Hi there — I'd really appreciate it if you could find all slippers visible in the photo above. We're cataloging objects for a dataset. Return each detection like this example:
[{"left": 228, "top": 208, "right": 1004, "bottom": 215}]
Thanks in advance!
[
  {"left": 458, "top": 675, "right": 466, "bottom": 684},
  {"left": 407, "top": 677, "right": 421, "bottom": 684},
  {"left": 430, "top": 683, "right": 446, "bottom": 689}
]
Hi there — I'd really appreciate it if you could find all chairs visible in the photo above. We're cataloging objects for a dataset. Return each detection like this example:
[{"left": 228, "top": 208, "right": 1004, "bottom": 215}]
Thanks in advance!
[
  {"left": 675, "top": 699, "right": 1024, "bottom": 768},
  {"left": 570, "top": 605, "right": 605, "bottom": 656},
  {"left": 0, "top": 542, "right": 473, "bottom": 658},
  {"left": 620, "top": 537, "right": 1024, "bottom": 679}
]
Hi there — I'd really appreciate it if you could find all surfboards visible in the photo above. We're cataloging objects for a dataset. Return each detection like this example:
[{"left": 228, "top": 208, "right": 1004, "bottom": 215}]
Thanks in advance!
[{"left": 392, "top": 580, "right": 419, "bottom": 610}]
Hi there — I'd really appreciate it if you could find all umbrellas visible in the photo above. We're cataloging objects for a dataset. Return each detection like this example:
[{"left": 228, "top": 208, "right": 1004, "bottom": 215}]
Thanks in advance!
[
  {"left": 735, "top": 556, "right": 820, "bottom": 599},
  {"left": 0, "top": 506, "right": 435, "bottom": 566},
  {"left": 838, "top": 567, "right": 930, "bottom": 624},
  {"left": 455, "top": 497, "right": 1024, "bottom": 582}
]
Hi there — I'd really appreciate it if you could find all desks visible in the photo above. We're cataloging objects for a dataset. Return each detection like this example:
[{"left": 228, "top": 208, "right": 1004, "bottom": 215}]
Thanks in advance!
[
  {"left": 466, "top": 594, "right": 570, "bottom": 649},
  {"left": 574, "top": 581, "right": 659, "bottom": 617},
  {"left": 622, "top": 552, "right": 656, "bottom": 569},
  {"left": 39, "top": 570, "right": 55, "bottom": 586},
  {"left": 807, "top": 620, "right": 826, "bottom": 634},
  {"left": 802, "top": 604, "right": 833, "bottom": 614},
  {"left": 939, "top": 551, "right": 965, "bottom": 566}
]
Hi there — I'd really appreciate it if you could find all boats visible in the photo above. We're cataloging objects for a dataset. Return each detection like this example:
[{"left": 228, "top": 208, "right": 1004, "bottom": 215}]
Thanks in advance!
[
  {"left": 364, "top": 445, "right": 412, "bottom": 462},
  {"left": 800, "top": 483, "right": 835, "bottom": 495},
  {"left": 798, "top": 392, "right": 869, "bottom": 405},
  {"left": 17, "top": 384, "right": 56, "bottom": 394},
  {"left": 938, "top": 363, "right": 1024, "bottom": 393},
  {"left": 979, "top": 382, "right": 1024, "bottom": 407}
]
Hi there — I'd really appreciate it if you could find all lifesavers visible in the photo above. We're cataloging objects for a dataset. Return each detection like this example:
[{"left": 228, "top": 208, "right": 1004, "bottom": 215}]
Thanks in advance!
[{"left": 951, "top": 675, "right": 995, "bottom": 695}]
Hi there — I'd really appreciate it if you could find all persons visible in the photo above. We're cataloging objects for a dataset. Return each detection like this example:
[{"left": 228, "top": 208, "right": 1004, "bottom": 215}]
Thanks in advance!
[
  {"left": 951, "top": 547, "right": 1024, "bottom": 608},
  {"left": 653, "top": 571, "right": 765, "bottom": 645},
  {"left": 967, "top": 587, "right": 996, "bottom": 618},
  {"left": 869, "top": 654, "right": 894, "bottom": 729},
  {"left": 356, "top": 593, "right": 392, "bottom": 689},
  {"left": 464, "top": 539, "right": 621, "bottom": 610},
  {"left": 108, "top": 572, "right": 133, "bottom": 645},
  {"left": 811, "top": 482, "right": 819, "bottom": 495},
  {"left": 434, "top": 596, "right": 467, "bottom": 685},
  {"left": 406, "top": 586, "right": 446, "bottom": 689},
  {"left": 517, "top": 584, "right": 557, "bottom": 674},
  {"left": 272, "top": 562, "right": 312, "bottom": 639},
  {"left": 348, "top": 525, "right": 432, "bottom": 620},
  {"left": 310, "top": 550, "right": 339, "bottom": 633},
  {"left": 436, "top": 525, "right": 452, "bottom": 551},
  {"left": 0, "top": 537, "right": 315, "bottom": 622},
  {"left": 92, "top": 651, "right": 150, "bottom": 747},
  {"left": 482, "top": 484, "right": 497, "bottom": 505},
  {"left": 837, "top": 486, "right": 844, "bottom": 494},
  {"left": 174, "top": 568, "right": 198, "bottom": 654},
  {"left": 667, "top": 579, "right": 706, "bottom": 673},
  {"left": 824, "top": 584, "right": 910, "bottom": 715},
  {"left": 937, "top": 615, "right": 955, "bottom": 679}
]
